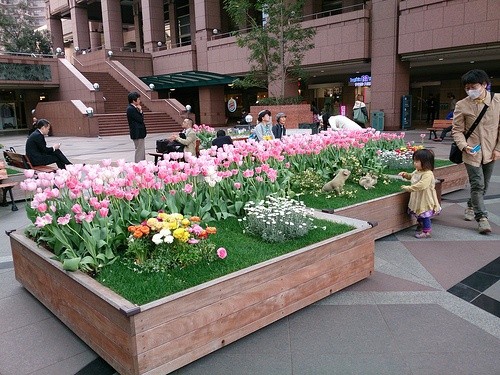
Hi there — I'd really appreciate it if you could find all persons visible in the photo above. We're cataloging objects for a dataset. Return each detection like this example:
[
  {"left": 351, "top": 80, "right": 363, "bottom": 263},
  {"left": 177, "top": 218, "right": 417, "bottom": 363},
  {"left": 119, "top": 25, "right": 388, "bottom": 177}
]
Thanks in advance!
[
  {"left": 254, "top": 109, "right": 275, "bottom": 142},
  {"left": 434, "top": 108, "right": 453, "bottom": 142},
  {"left": 127, "top": 92, "right": 146, "bottom": 163},
  {"left": 28, "top": 117, "right": 39, "bottom": 135},
  {"left": 399, "top": 149, "right": 442, "bottom": 238},
  {"left": 170, "top": 118, "right": 196, "bottom": 162},
  {"left": 323, "top": 113, "right": 362, "bottom": 130},
  {"left": 353, "top": 96, "right": 368, "bottom": 128},
  {"left": 25, "top": 119, "right": 72, "bottom": 170},
  {"left": 272, "top": 113, "right": 287, "bottom": 139},
  {"left": 452, "top": 70, "right": 500, "bottom": 233},
  {"left": 212, "top": 129, "right": 233, "bottom": 148},
  {"left": 450, "top": 95, "right": 456, "bottom": 110}
]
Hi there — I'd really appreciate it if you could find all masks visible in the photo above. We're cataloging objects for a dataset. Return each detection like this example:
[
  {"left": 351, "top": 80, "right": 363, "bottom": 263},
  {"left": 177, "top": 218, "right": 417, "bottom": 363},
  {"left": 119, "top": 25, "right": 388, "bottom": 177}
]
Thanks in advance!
[{"left": 467, "top": 86, "right": 485, "bottom": 99}]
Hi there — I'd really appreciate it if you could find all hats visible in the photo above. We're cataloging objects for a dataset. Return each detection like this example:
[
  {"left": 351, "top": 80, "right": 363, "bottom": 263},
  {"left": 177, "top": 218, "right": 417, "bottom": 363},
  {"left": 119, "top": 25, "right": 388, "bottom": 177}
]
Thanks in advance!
[{"left": 258, "top": 110, "right": 271, "bottom": 121}]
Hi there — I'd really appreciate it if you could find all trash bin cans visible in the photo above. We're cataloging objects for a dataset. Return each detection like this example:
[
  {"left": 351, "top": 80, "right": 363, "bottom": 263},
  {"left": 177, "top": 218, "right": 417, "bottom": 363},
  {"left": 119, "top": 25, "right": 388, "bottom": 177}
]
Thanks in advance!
[{"left": 371, "top": 111, "right": 385, "bottom": 131}]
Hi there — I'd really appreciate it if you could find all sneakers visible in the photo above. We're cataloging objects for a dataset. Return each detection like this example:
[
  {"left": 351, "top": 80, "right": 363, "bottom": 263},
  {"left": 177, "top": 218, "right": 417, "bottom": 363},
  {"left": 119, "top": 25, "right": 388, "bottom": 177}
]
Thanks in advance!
[
  {"left": 464, "top": 206, "right": 475, "bottom": 220},
  {"left": 479, "top": 217, "right": 492, "bottom": 232}
]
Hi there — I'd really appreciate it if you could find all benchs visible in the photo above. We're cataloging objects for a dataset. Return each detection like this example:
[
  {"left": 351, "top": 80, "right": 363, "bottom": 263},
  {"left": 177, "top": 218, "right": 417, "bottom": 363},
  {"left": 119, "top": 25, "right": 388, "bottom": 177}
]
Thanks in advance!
[
  {"left": 0, "top": 181, "right": 20, "bottom": 212},
  {"left": 426, "top": 119, "right": 453, "bottom": 140},
  {"left": 3, "top": 147, "right": 58, "bottom": 176},
  {"left": 232, "top": 137, "right": 249, "bottom": 144},
  {"left": 148, "top": 151, "right": 161, "bottom": 165}
]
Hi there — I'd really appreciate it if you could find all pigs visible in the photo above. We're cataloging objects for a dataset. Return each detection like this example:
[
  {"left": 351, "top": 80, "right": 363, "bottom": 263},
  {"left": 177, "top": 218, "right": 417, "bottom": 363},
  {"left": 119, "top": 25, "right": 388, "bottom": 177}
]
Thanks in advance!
[{"left": 320, "top": 167, "right": 352, "bottom": 195}]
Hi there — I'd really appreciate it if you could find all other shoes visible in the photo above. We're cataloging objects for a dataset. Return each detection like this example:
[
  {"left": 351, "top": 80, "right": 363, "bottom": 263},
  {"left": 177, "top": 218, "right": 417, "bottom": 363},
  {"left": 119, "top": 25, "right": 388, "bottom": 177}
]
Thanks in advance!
[
  {"left": 416, "top": 224, "right": 423, "bottom": 232},
  {"left": 415, "top": 232, "right": 430, "bottom": 238},
  {"left": 432, "top": 137, "right": 442, "bottom": 142}
]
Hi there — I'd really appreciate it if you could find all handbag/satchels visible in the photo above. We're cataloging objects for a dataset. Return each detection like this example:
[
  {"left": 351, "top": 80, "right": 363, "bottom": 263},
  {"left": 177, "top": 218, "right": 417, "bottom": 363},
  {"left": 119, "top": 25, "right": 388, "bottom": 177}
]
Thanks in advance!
[
  {"left": 450, "top": 143, "right": 463, "bottom": 164},
  {"left": 354, "top": 104, "right": 365, "bottom": 123},
  {"left": 156, "top": 140, "right": 168, "bottom": 152}
]
